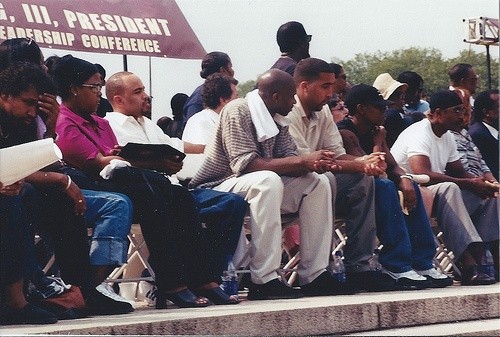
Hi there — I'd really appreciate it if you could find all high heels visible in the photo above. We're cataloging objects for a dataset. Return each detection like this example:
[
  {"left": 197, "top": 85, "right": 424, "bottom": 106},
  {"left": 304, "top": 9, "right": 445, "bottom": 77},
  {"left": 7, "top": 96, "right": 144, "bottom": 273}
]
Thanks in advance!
[
  {"left": 188, "top": 284, "right": 242, "bottom": 305},
  {"left": 155, "top": 288, "right": 210, "bottom": 310}
]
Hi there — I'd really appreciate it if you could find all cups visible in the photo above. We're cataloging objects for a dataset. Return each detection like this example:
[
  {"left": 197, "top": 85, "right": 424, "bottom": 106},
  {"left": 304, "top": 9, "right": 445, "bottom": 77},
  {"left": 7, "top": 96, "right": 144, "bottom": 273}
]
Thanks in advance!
[{"left": 118, "top": 282, "right": 137, "bottom": 299}]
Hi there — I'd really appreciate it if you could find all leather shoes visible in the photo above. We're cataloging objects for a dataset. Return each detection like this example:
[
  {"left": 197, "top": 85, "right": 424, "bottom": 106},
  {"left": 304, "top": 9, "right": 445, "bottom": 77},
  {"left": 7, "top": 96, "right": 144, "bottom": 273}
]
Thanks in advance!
[{"left": 460, "top": 271, "right": 497, "bottom": 286}]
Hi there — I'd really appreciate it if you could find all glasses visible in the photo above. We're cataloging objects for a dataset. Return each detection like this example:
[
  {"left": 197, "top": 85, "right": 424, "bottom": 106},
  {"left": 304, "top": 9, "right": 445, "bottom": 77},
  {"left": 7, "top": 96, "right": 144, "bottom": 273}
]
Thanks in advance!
[
  {"left": 81, "top": 84, "right": 102, "bottom": 94},
  {"left": 331, "top": 105, "right": 348, "bottom": 112},
  {"left": 336, "top": 75, "right": 347, "bottom": 81},
  {"left": 305, "top": 34, "right": 312, "bottom": 42},
  {"left": 26, "top": 36, "right": 36, "bottom": 45},
  {"left": 447, "top": 107, "right": 467, "bottom": 115},
  {"left": 465, "top": 74, "right": 480, "bottom": 81},
  {"left": 389, "top": 92, "right": 409, "bottom": 100}
]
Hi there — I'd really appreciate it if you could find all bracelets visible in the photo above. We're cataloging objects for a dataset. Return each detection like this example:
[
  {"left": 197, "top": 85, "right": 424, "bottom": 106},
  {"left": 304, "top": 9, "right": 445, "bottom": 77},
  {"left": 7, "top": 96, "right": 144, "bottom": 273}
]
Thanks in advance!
[
  {"left": 64, "top": 176, "right": 71, "bottom": 193},
  {"left": 400, "top": 175, "right": 413, "bottom": 180}
]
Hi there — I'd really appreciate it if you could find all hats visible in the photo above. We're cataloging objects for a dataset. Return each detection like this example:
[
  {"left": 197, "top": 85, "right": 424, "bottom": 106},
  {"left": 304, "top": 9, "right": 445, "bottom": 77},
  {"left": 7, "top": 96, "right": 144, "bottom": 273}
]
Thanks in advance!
[
  {"left": 344, "top": 83, "right": 394, "bottom": 107},
  {"left": 429, "top": 91, "right": 464, "bottom": 113},
  {"left": 372, "top": 73, "right": 409, "bottom": 101},
  {"left": 51, "top": 54, "right": 99, "bottom": 98}
]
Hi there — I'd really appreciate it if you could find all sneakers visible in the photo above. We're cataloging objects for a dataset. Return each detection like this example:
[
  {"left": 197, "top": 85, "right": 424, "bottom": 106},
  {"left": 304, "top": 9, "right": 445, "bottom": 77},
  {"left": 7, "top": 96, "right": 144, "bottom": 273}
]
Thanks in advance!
[
  {"left": 381, "top": 266, "right": 433, "bottom": 289},
  {"left": 415, "top": 268, "right": 454, "bottom": 288}
]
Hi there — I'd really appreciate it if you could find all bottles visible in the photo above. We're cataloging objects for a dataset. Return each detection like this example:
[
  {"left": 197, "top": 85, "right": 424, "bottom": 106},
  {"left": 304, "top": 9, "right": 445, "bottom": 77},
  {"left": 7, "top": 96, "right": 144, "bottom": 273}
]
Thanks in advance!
[
  {"left": 219, "top": 270, "right": 232, "bottom": 297},
  {"left": 482, "top": 245, "right": 496, "bottom": 279},
  {"left": 370, "top": 249, "right": 382, "bottom": 271},
  {"left": 226, "top": 255, "right": 239, "bottom": 301},
  {"left": 332, "top": 251, "right": 347, "bottom": 286}
]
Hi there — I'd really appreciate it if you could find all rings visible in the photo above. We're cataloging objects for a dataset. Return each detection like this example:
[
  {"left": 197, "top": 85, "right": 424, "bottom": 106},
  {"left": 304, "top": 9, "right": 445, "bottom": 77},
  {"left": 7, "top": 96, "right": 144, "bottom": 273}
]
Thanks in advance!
[{"left": 79, "top": 200, "right": 82, "bottom": 203}]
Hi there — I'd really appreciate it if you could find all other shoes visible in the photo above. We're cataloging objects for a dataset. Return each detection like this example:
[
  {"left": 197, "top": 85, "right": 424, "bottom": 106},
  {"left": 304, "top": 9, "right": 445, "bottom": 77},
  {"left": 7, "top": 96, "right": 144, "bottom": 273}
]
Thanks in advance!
[
  {"left": 0, "top": 302, "right": 59, "bottom": 325},
  {"left": 246, "top": 279, "right": 304, "bottom": 300},
  {"left": 96, "top": 282, "right": 136, "bottom": 309},
  {"left": 118, "top": 282, "right": 157, "bottom": 307},
  {"left": 79, "top": 285, "right": 133, "bottom": 316},
  {"left": 345, "top": 270, "right": 401, "bottom": 292},
  {"left": 301, "top": 271, "right": 356, "bottom": 296}
]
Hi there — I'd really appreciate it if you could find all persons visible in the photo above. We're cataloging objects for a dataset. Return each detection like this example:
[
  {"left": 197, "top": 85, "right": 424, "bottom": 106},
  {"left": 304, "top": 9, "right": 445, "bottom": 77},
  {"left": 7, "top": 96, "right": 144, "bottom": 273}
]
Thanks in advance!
[
  {"left": 0, "top": 38, "right": 245, "bottom": 325},
  {"left": 286, "top": 59, "right": 500, "bottom": 292},
  {"left": 188, "top": 69, "right": 340, "bottom": 297},
  {"left": 271, "top": 21, "right": 312, "bottom": 75}
]
